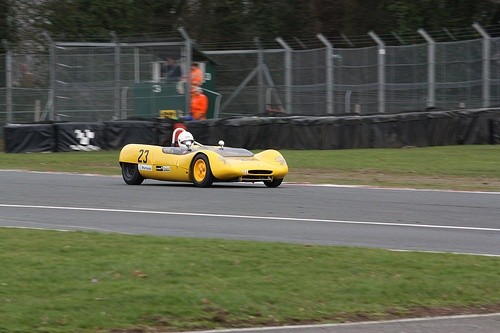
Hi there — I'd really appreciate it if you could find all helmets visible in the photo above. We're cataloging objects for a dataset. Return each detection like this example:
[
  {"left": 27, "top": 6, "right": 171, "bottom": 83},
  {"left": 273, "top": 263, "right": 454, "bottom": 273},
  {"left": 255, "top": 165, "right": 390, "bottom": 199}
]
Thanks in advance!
[{"left": 177, "top": 131, "right": 194, "bottom": 149}]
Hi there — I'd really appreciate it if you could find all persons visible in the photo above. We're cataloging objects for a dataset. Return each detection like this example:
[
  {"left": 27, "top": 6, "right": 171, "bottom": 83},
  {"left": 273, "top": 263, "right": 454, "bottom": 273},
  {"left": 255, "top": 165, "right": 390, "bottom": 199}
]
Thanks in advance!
[
  {"left": 190, "top": 61, "right": 203, "bottom": 88},
  {"left": 160, "top": 54, "right": 181, "bottom": 76},
  {"left": 178, "top": 130, "right": 195, "bottom": 151},
  {"left": 188, "top": 87, "right": 209, "bottom": 119}
]
